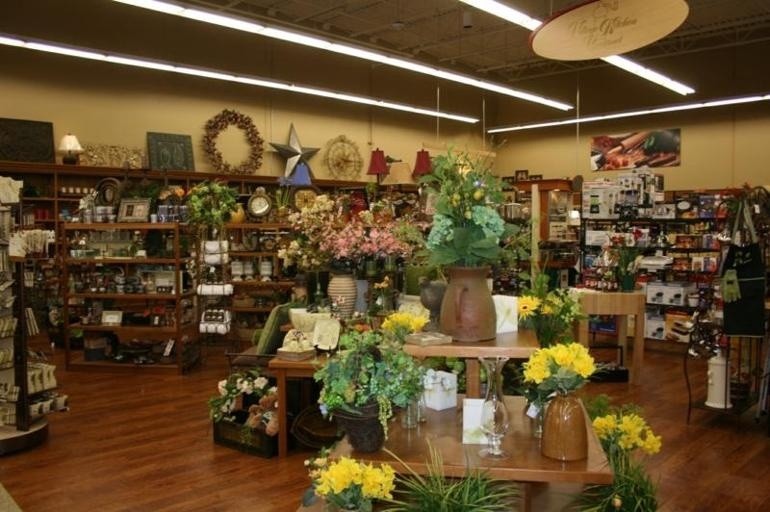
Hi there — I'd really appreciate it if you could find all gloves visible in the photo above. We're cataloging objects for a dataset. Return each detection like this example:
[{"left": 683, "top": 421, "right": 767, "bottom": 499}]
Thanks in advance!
[{"left": 721, "top": 269, "right": 742, "bottom": 304}]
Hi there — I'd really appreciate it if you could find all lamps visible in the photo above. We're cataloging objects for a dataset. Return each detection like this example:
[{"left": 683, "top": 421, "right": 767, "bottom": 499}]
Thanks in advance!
[
  {"left": 366, "top": 148, "right": 390, "bottom": 183},
  {"left": 411, "top": 149, "right": 433, "bottom": 186},
  {"left": 0, "top": 0, "right": 770, "bottom": 135},
  {"left": 58, "top": 133, "right": 83, "bottom": 165}
]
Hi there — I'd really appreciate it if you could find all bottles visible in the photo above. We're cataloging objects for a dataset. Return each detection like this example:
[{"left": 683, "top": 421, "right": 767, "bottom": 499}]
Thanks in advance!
[{"left": 478, "top": 354, "right": 515, "bottom": 460}]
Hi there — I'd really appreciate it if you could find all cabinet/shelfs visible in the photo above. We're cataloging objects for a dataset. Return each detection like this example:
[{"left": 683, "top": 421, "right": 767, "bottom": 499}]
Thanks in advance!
[{"left": 0, "top": 159, "right": 770, "bottom": 512}]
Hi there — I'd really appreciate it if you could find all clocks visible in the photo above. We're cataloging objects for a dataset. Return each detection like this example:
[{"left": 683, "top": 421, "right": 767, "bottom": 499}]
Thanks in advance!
[{"left": 322, "top": 135, "right": 364, "bottom": 181}]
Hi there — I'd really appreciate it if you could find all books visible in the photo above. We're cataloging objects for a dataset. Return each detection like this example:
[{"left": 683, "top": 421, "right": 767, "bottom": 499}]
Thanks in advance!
[
  {"left": 668, "top": 195, "right": 731, "bottom": 272},
  {"left": 1, "top": 208, "right": 68, "bottom": 425}
]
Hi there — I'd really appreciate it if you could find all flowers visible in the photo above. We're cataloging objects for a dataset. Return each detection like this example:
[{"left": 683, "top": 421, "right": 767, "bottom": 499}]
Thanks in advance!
[{"left": 200, "top": 108, "right": 265, "bottom": 176}]
